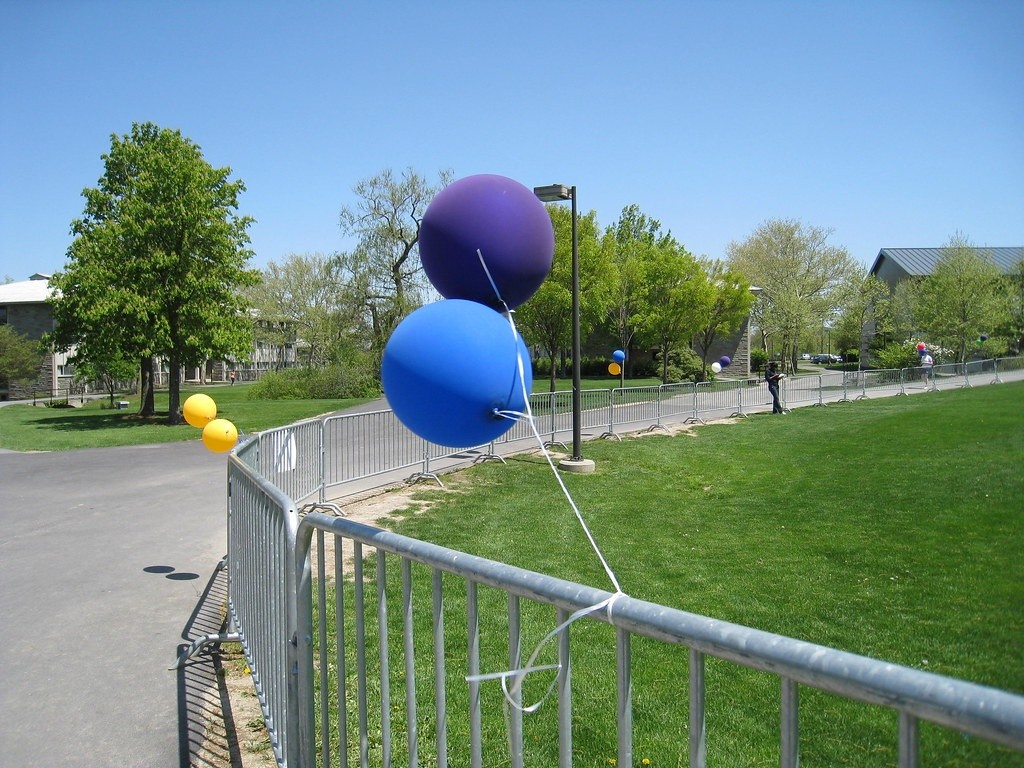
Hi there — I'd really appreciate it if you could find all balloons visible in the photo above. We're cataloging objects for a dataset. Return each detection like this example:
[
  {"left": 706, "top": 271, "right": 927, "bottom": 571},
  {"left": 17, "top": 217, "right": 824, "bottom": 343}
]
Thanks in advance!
[
  {"left": 381, "top": 298, "right": 533, "bottom": 448},
  {"left": 612, "top": 349, "right": 625, "bottom": 363},
  {"left": 607, "top": 362, "right": 621, "bottom": 376},
  {"left": 917, "top": 343, "right": 927, "bottom": 356},
  {"left": 419, "top": 174, "right": 555, "bottom": 314},
  {"left": 202, "top": 418, "right": 238, "bottom": 454},
  {"left": 719, "top": 356, "right": 730, "bottom": 367},
  {"left": 184, "top": 394, "right": 218, "bottom": 429},
  {"left": 711, "top": 362, "right": 722, "bottom": 374},
  {"left": 975, "top": 334, "right": 988, "bottom": 345}
]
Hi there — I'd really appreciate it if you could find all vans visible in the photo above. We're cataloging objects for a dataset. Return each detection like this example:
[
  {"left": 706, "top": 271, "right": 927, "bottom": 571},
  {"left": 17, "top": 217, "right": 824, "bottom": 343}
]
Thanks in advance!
[{"left": 802, "top": 354, "right": 811, "bottom": 360}]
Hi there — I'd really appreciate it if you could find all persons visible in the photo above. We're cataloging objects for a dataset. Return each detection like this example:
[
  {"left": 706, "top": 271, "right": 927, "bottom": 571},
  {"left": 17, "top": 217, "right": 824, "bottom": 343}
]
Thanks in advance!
[
  {"left": 765, "top": 362, "right": 787, "bottom": 415},
  {"left": 231, "top": 370, "right": 235, "bottom": 386},
  {"left": 920, "top": 351, "right": 933, "bottom": 389}
]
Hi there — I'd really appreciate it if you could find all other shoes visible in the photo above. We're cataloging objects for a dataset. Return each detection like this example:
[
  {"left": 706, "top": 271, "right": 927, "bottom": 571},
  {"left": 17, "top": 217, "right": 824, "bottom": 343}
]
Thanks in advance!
[
  {"left": 924, "top": 386, "right": 927, "bottom": 389},
  {"left": 781, "top": 411, "right": 786, "bottom": 415}
]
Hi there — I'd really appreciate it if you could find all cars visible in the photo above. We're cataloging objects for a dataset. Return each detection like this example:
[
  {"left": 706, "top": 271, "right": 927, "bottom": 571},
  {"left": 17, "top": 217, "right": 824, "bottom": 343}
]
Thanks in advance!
[{"left": 813, "top": 353, "right": 842, "bottom": 364}]
